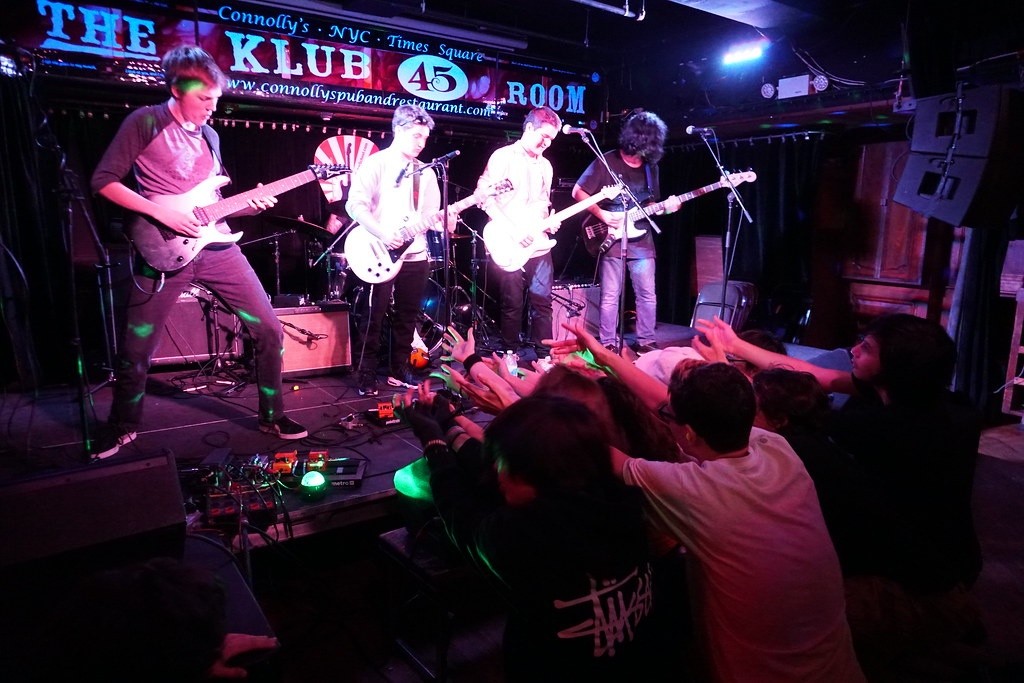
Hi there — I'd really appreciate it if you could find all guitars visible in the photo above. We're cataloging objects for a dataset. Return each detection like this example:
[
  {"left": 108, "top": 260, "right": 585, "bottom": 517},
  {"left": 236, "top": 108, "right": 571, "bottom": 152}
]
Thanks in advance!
[
  {"left": 482, "top": 183, "right": 624, "bottom": 275},
  {"left": 343, "top": 176, "right": 515, "bottom": 285},
  {"left": 124, "top": 161, "right": 353, "bottom": 277},
  {"left": 580, "top": 167, "right": 757, "bottom": 260}
]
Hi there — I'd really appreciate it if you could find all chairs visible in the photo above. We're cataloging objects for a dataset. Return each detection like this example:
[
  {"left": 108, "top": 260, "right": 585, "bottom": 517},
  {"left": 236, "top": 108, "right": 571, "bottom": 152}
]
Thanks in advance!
[{"left": 688, "top": 279, "right": 756, "bottom": 333}]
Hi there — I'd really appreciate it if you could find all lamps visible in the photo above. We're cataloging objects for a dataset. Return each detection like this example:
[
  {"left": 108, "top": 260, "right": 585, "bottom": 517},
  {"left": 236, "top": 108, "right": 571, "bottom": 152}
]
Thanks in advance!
[{"left": 761, "top": 74, "right": 829, "bottom": 99}]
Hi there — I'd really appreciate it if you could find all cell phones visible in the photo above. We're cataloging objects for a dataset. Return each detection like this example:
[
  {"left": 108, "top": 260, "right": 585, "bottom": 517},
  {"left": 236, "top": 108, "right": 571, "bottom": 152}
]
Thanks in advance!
[{"left": 168, "top": 283, "right": 244, "bottom": 386}]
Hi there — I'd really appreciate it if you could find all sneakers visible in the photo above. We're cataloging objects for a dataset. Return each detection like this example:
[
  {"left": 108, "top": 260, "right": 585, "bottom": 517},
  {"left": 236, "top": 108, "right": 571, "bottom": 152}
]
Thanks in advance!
[
  {"left": 359, "top": 379, "right": 378, "bottom": 396},
  {"left": 386, "top": 365, "right": 423, "bottom": 390},
  {"left": 257, "top": 416, "right": 308, "bottom": 440},
  {"left": 88, "top": 427, "right": 140, "bottom": 460}
]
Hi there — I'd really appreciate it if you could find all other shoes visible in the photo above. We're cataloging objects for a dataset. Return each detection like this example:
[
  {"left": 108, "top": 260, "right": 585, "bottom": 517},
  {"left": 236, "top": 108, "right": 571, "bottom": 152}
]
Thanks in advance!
[
  {"left": 605, "top": 344, "right": 618, "bottom": 354},
  {"left": 533, "top": 347, "right": 550, "bottom": 360},
  {"left": 632, "top": 342, "right": 662, "bottom": 354}
]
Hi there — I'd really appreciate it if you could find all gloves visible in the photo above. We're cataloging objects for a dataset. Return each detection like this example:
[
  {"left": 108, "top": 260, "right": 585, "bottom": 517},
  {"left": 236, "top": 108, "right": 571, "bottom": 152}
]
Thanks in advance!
[
  {"left": 403, "top": 401, "right": 446, "bottom": 446},
  {"left": 432, "top": 397, "right": 460, "bottom": 435}
]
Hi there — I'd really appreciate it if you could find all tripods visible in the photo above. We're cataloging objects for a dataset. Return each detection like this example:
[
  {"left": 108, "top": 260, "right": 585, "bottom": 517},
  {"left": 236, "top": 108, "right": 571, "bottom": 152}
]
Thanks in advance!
[
  {"left": 452, "top": 222, "right": 503, "bottom": 349},
  {"left": 70, "top": 262, "right": 120, "bottom": 403}
]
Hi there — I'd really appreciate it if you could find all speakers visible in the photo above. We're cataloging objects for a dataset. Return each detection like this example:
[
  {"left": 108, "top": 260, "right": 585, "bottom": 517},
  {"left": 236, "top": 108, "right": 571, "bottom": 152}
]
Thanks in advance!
[
  {"left": 151, "top": 282, "right": 244, "bottom": 367},
  {"left": 273, "top": 304, "right": 353, "bottom": 382},
  {"left": 526, "top": 281, "right": 605, "bottom": 341},
  {"left": 0, "top": 448, "right": 188, "bottom": 585}
]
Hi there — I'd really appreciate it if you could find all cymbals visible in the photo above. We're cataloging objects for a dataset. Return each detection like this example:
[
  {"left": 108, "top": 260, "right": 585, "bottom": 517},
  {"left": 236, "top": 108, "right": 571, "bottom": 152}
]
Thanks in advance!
[
  {"left": 260, "top": 212, "right": 336, "bottom": 240},
  {"left": 449, "top": 233, "right": 472, "bottom": 239}
]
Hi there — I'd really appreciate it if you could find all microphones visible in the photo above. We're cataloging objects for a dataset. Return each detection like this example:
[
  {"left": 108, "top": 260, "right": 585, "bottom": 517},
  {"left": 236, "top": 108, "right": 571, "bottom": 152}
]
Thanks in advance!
[
  {"left": 436, "top": 325, "right": 447, "bottom": 333},
  {"left": 562, "top": 124, "right": 591, "bottom": 134},
  {"left": 307, "top": 337, "right": 327, "bottom": 342},
  {"left": 392, "top": 163, "right": 410, "bottom": 188},
  {"left": 686, "top": 126, "right": 713, "bottom": 134},
  {"left": 566, "top": 307, "right": 585, "bottom": 318}
]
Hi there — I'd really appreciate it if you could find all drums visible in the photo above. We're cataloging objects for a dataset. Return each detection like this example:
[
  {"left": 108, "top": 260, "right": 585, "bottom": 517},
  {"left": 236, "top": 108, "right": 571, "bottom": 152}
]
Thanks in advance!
[
  {"left": 424, "top": 229, "right": 451, "bottom": 274},
  {"left": 308, "top": 252, "right": 355, "bottom": 303},
  {"left": 351, "top": 275, "right": 454, "bottom": 357}
]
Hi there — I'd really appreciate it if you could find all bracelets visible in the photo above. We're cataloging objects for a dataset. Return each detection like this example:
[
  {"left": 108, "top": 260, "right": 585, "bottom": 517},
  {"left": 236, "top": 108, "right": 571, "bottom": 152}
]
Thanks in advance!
[
  {"left": 452, "top": 409, "right": 461, "bottom": 417},
  {"left": 463, "top": 353, "right": 482, "bottom": 374}
]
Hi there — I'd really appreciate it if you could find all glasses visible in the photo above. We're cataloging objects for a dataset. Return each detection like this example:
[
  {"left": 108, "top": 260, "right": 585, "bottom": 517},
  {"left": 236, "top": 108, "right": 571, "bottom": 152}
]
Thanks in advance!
[
  {"left": 725, "top": 354, "right": 747, "bottom": 364},
  {"left": 399, "top": 115, "right": 429, "bottom": 125},
  {"left": 658, "top": 403, "right": 683, "bottom": 425}
]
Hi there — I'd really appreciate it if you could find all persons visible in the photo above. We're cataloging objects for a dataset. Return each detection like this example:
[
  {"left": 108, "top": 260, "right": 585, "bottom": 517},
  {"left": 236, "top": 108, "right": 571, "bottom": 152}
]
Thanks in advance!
[
  {"left": 1, "top": 612, "right": 279, "bottom": 683},
  {"left": 89, "top": 45, "right": 307, "bottom": 461},
  {"left": 394, "top": 314, "right": 986, "bottom": 683},
  {"left": 572, "top": 112, "right": 667, "bottom": 357},
  {"left": 475, "top": 106, "right": 562, "bottom": 372},
  {"left": 345, "top": 105, "right": 458, "bottom": 394}
]
